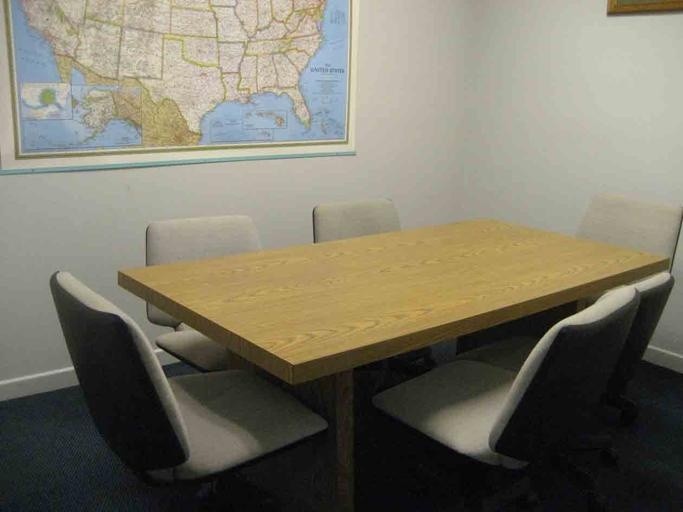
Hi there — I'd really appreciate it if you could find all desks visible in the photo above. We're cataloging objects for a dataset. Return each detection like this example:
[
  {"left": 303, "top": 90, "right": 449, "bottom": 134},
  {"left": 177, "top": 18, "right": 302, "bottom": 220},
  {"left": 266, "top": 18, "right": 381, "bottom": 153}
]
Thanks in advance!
[{"left": 120, "top": 220, "right": 669, "bottom": 512}]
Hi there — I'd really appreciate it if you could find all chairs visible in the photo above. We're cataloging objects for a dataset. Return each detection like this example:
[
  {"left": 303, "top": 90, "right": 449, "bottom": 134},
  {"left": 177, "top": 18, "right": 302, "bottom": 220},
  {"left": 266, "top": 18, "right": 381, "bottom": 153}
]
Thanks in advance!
[
  {"left": 49, "top": 271, "right": 328, "bottom": 512},
  {"left": 456, "top": 272, "right": 675, "bottom": 466},
  {"left": 144, "top": 216, "right": 265, "bottom": 371},
  {"left": 313, "top": 198, "right": 402, "bottom": 245},
  {"left": 571, "top": 188, "right": 683, "bottom": 314},
  {"left": 370, "top": 286, "right": 640, "bottom": 511}
]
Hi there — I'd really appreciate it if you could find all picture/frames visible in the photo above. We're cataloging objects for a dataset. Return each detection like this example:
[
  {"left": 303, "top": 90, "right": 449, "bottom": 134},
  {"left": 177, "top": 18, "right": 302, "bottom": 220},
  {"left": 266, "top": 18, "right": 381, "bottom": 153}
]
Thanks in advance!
[{"left": 607, "top": 0, "right": 683, "bottom": 16}]
